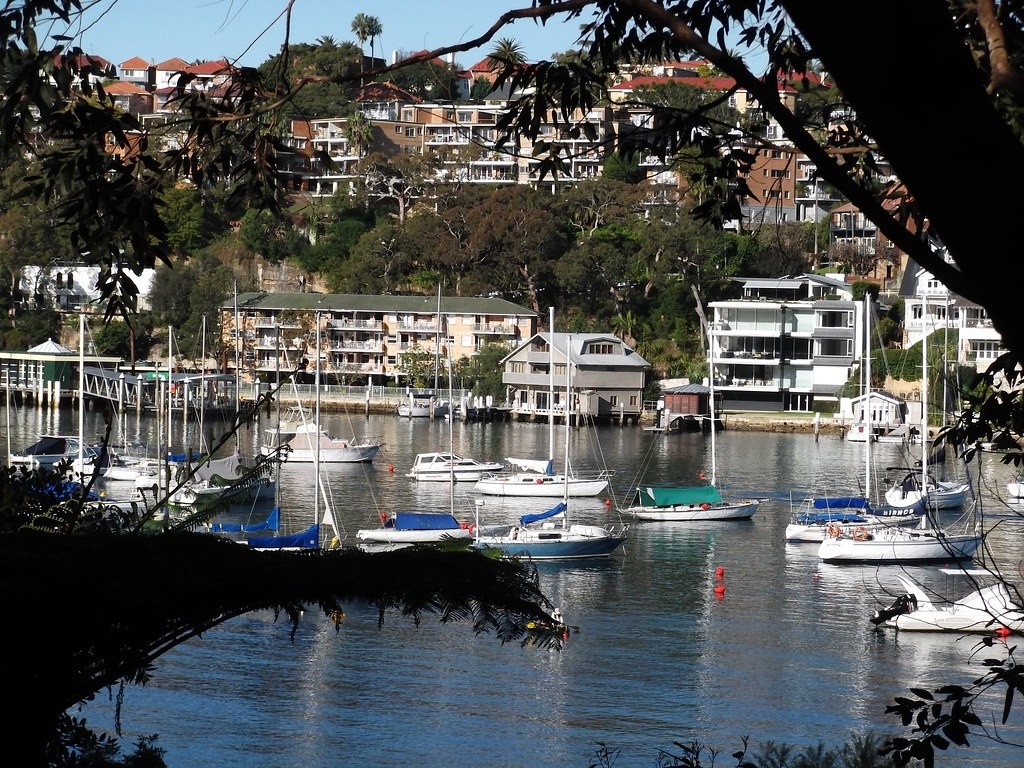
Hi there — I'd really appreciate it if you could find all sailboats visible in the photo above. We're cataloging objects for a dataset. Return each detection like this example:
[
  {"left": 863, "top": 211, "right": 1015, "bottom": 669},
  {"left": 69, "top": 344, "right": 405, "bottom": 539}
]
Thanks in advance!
[
  {"left": 5, "top": 280, "right": 522, "bottom": 550},
  {"left": 470, "top": 304, "right": 617, "bottom": 496},
  {"left": 785, "top": 290, "right": 923, "bottom": 545},
  {"left": 885, "top": 293, "right": 972, "bottom": 512},
  {"left": 817, "top": 293, "right": 984, "bottom": 566},
  {"left": 615, "top": 327, "right": 759, "bottom": 522}
]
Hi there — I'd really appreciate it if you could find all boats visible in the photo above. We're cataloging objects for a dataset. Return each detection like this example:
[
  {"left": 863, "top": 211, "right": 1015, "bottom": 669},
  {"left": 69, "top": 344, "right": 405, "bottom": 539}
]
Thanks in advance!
[
  {"left": 260, "top": 404, "right": 386, "bottom": 464},
  {"left": 869, "top": 572, "right": 1023, "bottom": 633},
  {"left": 405, "top": 451, "right": 506, "bottom": 484}
]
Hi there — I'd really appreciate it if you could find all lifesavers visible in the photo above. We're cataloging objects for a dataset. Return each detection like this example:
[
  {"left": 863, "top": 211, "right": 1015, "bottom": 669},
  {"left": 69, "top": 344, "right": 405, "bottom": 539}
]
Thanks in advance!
[
  {"left": 853, "top": 527, "right": 867, "bottom": 540},
  {"left": 828, "top": 523, "right": 841, "bottom": 537},
  {"left": 469, "top": 523, "right": 480, "bottom": 537}
]
